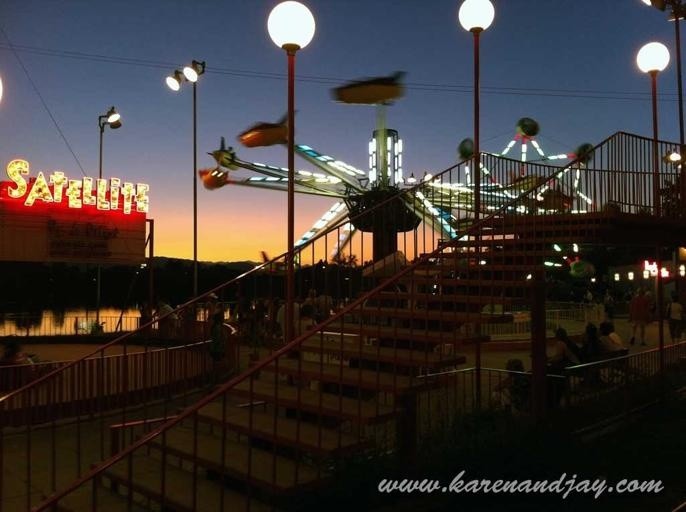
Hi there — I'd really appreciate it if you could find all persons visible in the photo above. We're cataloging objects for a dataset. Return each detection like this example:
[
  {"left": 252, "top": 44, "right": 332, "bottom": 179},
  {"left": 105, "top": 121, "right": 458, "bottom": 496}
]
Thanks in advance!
[{"left": 154, "top": 286, "right": 686, "bottom": 421}]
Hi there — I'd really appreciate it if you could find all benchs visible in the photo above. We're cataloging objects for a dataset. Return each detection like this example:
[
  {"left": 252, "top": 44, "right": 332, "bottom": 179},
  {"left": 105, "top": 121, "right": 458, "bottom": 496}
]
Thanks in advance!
[{"left": 579, "top": 348, "right": 628, "bottom": 388}]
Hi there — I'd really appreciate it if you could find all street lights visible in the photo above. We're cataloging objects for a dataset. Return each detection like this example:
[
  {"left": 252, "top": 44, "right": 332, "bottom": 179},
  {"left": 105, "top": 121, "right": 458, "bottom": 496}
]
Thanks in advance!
[
  {"left": 453, "top": 0, "right": 500, "bottom": 411},
  {"left": 95, "top": 102, "right": 124, "bottom": 336},
  {"left": 261, "top": 0, "right": 316, "bottom": 355},
  {"left": 641, "top": 1, "right": 686, "bottom": 172},
  {"left": 665, "top": 146, "right": 682, "bottom": 202},
  {"left": 165, "top": 59, "right": 206, "bottom": 319},
  {"left": 632, "top": 42, "right": 674, "bottom": 369}
]
